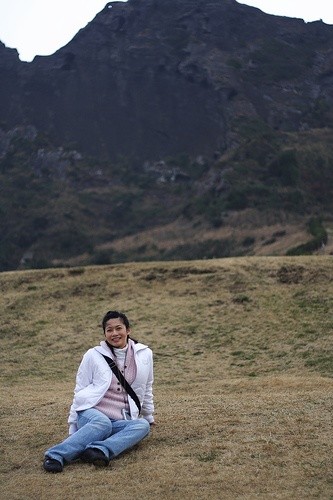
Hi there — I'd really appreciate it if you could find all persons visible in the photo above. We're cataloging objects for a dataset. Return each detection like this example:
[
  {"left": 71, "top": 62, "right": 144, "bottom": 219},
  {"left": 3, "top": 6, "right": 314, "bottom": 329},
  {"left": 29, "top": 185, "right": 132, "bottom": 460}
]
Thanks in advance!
[{"left": 43, "top": 310, "right": 159, "bottom": 474}]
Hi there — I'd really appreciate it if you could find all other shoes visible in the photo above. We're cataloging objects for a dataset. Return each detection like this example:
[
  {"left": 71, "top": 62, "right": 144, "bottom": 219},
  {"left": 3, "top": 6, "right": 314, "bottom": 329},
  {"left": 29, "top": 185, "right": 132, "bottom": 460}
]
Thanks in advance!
[
  {"left": 81, "top": 448, "right": 109, "bottom": 467},
  {"left": 43, "top": 455, "right": 62, "bottom": 472}
]
